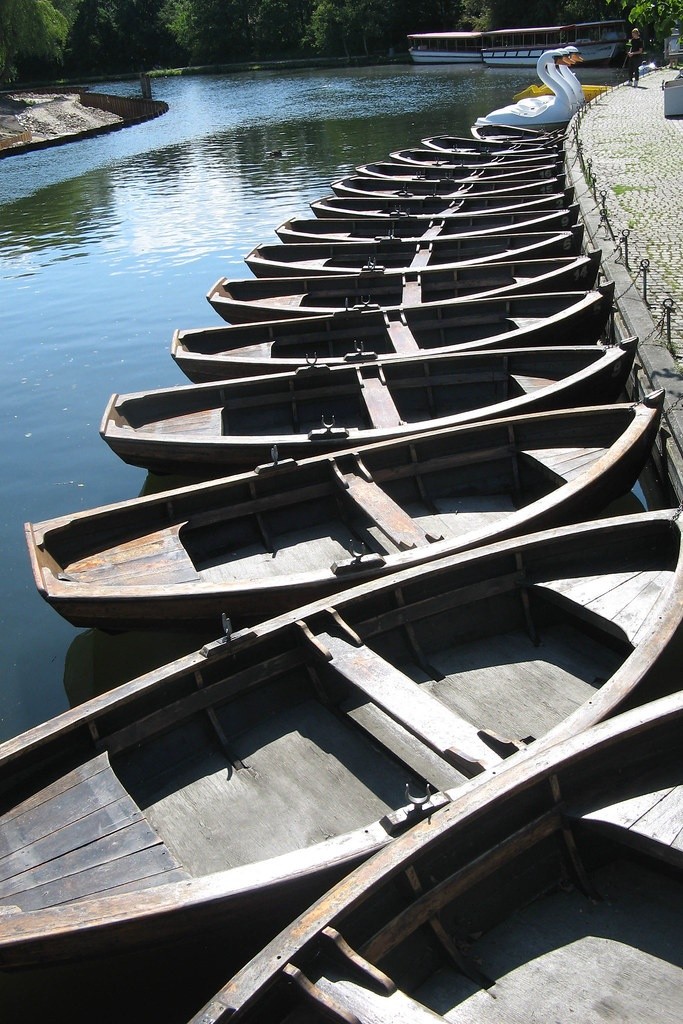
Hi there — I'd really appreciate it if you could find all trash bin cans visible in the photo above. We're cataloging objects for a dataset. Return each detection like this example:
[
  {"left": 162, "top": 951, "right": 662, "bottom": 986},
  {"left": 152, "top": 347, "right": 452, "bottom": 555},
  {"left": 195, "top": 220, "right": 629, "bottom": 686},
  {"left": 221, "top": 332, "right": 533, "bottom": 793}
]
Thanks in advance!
[{"left": 663, "top": 80, "right": 683, "bottom": 118}]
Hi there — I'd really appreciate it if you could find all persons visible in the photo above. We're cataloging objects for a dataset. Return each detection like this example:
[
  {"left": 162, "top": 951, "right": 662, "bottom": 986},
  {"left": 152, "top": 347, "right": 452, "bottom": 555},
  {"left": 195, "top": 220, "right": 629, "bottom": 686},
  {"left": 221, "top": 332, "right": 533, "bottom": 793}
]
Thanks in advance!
[
  {"left": 623, "top": 28, "right": 643, "bottom": 87},
  {"left": 667, "top": 37, "right": 680, "bottom": 70}
]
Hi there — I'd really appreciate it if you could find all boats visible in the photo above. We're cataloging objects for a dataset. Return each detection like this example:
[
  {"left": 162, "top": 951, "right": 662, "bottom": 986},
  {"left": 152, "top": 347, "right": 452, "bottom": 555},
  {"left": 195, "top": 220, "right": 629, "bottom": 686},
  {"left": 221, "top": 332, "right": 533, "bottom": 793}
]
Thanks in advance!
[{"left": 0, "top": 18, "right": 683, "bottom": 1024}]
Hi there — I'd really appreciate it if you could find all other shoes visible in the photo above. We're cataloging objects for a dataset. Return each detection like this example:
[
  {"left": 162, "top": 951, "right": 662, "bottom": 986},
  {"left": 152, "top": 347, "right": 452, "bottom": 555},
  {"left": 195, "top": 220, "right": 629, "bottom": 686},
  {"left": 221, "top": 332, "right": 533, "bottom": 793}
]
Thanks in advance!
[
  {"left": 627, "top": 81, "right": 632, "bottom": 85},
  {"left": 634, "top": 81, "right": 638, "bottom": 87}
]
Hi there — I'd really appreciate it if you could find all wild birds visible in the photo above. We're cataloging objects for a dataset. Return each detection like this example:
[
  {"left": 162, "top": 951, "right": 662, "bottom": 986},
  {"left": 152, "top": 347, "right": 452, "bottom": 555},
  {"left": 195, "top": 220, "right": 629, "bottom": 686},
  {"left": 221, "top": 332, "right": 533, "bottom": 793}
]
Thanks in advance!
[{"left": 270, "top": 149, "right": 282, "bottom": 155}]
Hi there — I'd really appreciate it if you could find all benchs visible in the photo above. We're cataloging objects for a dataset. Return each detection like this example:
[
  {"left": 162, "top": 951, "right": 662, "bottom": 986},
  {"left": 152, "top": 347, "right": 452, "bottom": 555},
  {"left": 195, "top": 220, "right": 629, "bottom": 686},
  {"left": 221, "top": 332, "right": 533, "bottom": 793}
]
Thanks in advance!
[
  {"left": 447, "top": 183, "right": 474, "bottom": 196},
  {"left": 483, "top": 157, "right": 505, "bottom": 165},
  {"left": 381, "top": 309, "right": 424, "bottom": 354},
  {"left": 329, "top": 452, "right": 443, "bottom": 556},
  {"left": 501, "top": 144, "right": 522, "bottom": 152},
  {"left": 355, "top": 362, "right": 406, "bottom": 433},
  {"left": 298, "top": 608, "right": 522, "bottom": 779},
  {"left": 400, "top": 272, "right": 422, "bottom": 306},
  {"left": 433, "top": 199, "right": 464, "bottom": 215},
  {"left": 409, "top": 243, "right": 433, "bottom": 267},
  {"left": 420, "top": 220, "right": 445, "bottom": 237},
  {"left": 462, "top": 170, "right": 485, "bottom": 180}
]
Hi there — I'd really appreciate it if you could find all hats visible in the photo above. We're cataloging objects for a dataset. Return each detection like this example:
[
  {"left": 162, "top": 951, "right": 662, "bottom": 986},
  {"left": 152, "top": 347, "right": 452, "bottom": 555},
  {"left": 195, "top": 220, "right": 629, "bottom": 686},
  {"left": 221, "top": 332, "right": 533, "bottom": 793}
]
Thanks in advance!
[{"left": 633, "top": 28, "right": 640, "bottom": 35}]
